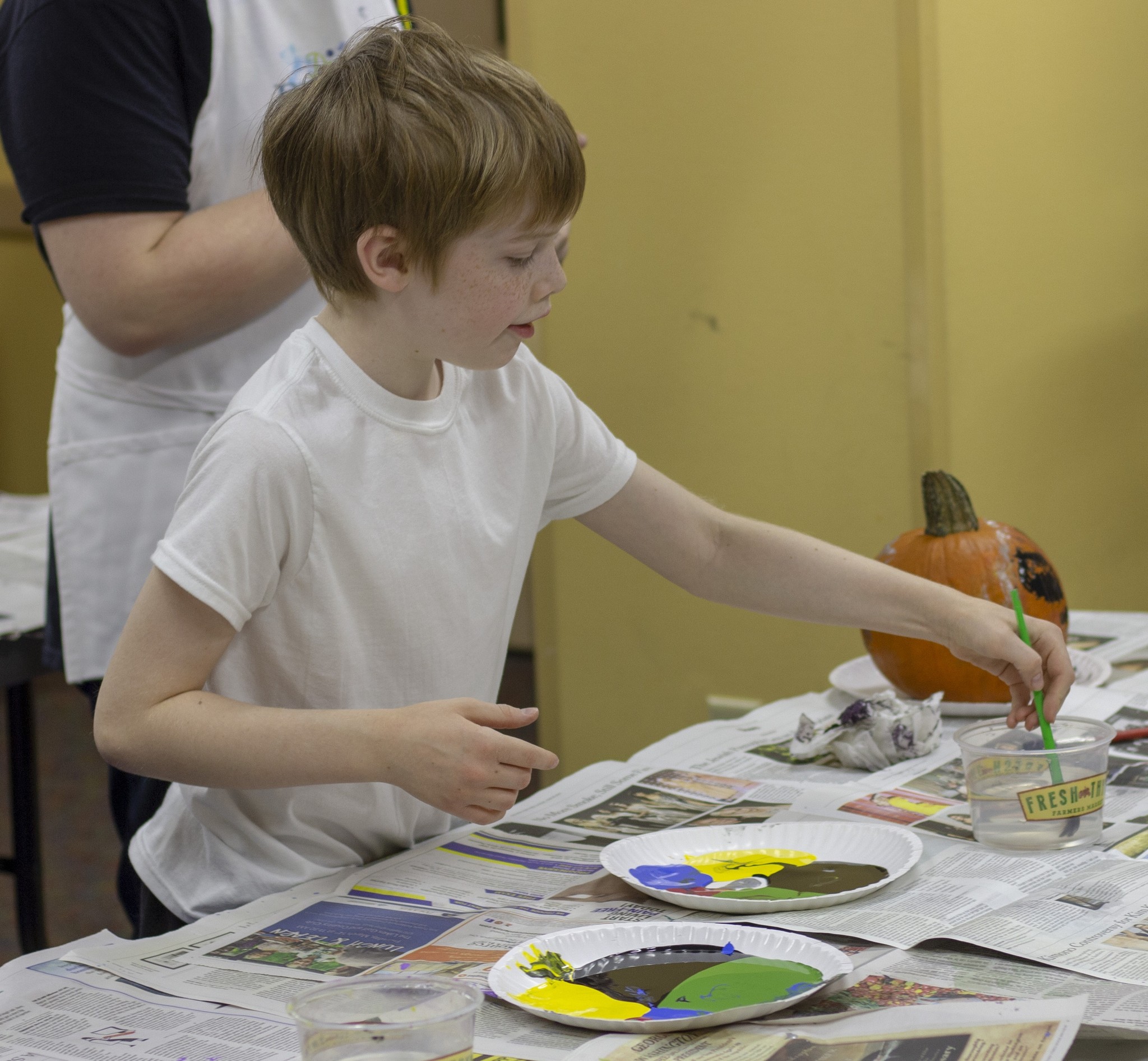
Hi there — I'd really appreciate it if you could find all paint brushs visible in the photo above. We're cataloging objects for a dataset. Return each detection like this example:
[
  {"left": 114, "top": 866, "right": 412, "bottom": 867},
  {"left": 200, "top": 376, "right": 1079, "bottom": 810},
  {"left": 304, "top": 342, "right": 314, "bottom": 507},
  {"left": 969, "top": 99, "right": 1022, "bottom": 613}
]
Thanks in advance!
[{"left": 1009, "top": 586, "right": 1081, "bottom": 841}]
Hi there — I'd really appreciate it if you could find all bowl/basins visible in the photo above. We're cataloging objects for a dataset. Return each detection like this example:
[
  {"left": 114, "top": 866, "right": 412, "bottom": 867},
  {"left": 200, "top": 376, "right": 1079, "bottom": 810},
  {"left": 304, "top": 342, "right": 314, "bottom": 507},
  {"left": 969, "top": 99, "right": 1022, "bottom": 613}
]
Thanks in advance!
[{"left": 951, "top": 715, "right": 1117, "bottom": 850}]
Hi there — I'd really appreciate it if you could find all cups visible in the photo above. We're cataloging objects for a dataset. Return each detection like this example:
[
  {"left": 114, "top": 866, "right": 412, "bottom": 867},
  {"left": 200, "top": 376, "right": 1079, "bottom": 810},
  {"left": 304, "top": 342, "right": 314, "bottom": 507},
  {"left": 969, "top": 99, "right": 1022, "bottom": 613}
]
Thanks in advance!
[{"left": 287, "top": 972, "right": 485, "bottom": 1061}]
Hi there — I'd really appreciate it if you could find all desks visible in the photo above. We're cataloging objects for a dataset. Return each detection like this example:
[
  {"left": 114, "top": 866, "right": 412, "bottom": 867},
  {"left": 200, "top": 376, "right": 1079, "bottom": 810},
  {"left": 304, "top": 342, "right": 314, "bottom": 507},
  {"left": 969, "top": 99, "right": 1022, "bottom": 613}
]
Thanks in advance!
[{"left": 0, "top": 612, "right": 1148, "bottom": 1060}]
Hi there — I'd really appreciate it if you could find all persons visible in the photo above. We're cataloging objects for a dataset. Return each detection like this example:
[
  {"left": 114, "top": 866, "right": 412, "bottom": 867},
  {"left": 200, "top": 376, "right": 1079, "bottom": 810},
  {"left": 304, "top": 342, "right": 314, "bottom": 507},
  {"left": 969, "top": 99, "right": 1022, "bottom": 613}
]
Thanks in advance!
[
  {"left": 0, "top": 0, "right": 404, "bottom": 934},
  {"left": 91, "top": 24, "right": 1077, "bottom": 944}
]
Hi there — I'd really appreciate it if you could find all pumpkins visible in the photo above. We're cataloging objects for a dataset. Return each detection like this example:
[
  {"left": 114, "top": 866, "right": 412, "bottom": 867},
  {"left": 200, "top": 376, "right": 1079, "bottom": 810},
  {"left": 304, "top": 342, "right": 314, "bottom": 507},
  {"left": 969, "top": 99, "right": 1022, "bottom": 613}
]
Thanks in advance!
[{"left": 862, "top": 470, "right": 1068, "bottom": 703}]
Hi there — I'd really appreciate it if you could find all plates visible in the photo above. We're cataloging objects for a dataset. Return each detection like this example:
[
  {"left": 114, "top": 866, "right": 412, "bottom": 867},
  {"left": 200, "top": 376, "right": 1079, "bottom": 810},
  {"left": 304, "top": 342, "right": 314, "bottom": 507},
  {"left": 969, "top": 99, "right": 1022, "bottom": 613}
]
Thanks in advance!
[
  {"left": 828, "top": 646, "right": 1112, "bottom": 716},
  {"left": 487, "top": 922, "right": 852, "bottom": 1035},
  {"left": 596, "top": 821, "right": 923, "bottom": 914}
]
